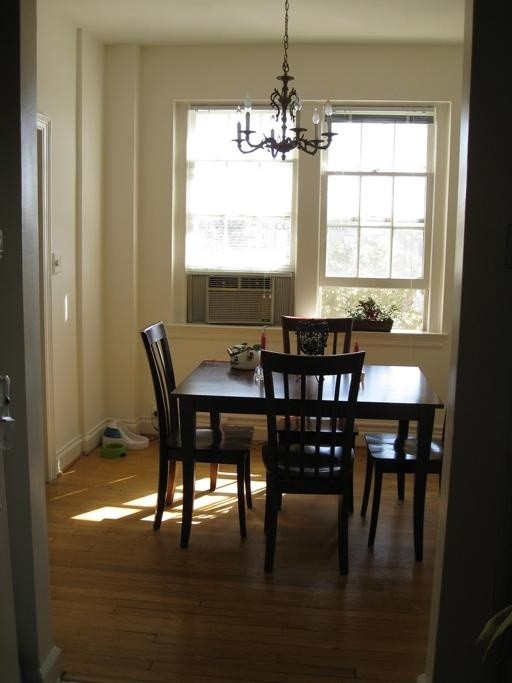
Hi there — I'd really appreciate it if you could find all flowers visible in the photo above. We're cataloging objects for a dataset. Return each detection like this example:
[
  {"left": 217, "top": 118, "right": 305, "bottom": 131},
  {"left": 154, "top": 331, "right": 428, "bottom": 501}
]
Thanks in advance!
[{"left": 345, "top": 297, "right": 404, "bottom": 321}]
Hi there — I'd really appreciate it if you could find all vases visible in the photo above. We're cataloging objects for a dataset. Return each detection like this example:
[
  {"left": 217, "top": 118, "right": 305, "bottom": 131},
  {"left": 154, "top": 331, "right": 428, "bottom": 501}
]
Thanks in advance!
[{"left": 347, "top": 319, "right": 395, "bottom": 331}]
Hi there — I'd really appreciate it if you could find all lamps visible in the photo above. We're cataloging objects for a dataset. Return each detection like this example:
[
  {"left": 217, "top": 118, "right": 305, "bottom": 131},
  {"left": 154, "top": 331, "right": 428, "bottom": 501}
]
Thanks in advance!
[{"left": 232, "top": 0, "right": 338, "bottom": 160}]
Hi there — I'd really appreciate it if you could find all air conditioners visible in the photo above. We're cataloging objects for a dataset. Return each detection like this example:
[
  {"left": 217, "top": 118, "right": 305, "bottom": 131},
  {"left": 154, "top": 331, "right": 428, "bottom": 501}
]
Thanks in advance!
[{"left": 188, "top": 272, "right": 292, "bottom": 326}]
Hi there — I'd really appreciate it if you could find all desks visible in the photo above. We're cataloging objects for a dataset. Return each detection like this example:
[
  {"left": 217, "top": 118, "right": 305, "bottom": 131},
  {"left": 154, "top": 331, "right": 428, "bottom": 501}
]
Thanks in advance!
[{"left": 168, "top": 360, "right": 444, "bottom": 561}]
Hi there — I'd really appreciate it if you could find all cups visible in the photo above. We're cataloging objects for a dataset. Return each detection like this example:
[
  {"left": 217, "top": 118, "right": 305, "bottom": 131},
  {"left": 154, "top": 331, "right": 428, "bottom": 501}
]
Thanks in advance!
[{"left": 254, "top": 366, "right": 264, "bottom": 382}]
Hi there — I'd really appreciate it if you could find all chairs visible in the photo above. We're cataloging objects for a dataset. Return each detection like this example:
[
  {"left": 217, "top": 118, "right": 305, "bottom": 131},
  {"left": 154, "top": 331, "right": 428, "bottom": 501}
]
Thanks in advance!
[
  {"left": 281, "top": 315, "right": 353, "bottom": 512},
  {"left": 361, "top": 409, "right": 447, "bottom": 545},
  {"left": 261, "top": 350, "right": 365, "bottom": 576},
  {"left": 141, "top": 321, "right": 255, "bottom": 538}
]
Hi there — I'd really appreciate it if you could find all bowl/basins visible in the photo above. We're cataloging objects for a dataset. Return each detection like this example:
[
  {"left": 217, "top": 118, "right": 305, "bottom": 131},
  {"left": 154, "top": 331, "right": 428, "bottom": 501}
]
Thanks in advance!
[{"left": 102, "top": 443, "right": 125, "bottom": 458}]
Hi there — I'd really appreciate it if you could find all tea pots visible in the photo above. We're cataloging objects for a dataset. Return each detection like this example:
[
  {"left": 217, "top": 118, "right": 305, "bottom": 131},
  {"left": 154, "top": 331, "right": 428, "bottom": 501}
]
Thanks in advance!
[{"left": 229, "top": 340, "right": 261, "bottom": 370}]
